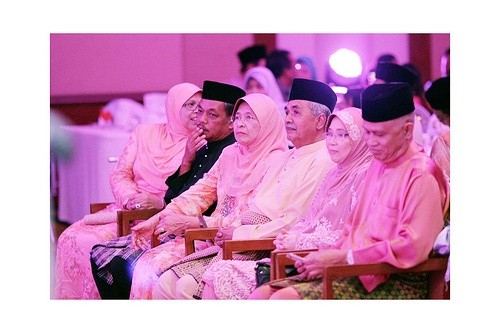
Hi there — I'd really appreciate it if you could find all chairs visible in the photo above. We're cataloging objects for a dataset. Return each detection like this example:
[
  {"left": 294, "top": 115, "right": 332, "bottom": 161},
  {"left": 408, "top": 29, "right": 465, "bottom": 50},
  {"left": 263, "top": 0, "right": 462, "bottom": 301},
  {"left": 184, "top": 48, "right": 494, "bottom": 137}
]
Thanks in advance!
[
  {"left": 89, "top": 201, "right": 450, "bottom": 300},
  {"left": 96, "top": 99, "right": 145, "bottom": 125}
]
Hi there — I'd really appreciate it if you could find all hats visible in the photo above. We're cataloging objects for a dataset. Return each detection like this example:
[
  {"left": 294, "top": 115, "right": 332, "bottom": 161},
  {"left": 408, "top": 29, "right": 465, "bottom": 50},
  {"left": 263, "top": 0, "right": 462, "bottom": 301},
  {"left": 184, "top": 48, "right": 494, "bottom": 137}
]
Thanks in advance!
[
  {"left": 288, "top": 78, "right": 337, "bottom": 114},
  {"left": 361, "top": 83, "right": 415, "bottom": 123},
  {"left": 375, "top": 62, "right": 418, "bottom": 94},
  {"left": 236, "top": 43, "right": 267, "bottom": 66},
  {"left": 201, "top": 80, "right": 245, "bottom": 105}
]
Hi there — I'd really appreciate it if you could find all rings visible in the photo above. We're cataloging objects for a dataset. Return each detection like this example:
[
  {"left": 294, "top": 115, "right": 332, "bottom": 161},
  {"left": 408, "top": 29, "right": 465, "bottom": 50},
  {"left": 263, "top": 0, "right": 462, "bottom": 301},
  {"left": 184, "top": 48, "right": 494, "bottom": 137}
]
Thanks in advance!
[
  {"left": 135, "top": 204, "right": 141, "bottom": 209},
  {"left": 161, "top": 227, "right": 164, "bottom": 232}
]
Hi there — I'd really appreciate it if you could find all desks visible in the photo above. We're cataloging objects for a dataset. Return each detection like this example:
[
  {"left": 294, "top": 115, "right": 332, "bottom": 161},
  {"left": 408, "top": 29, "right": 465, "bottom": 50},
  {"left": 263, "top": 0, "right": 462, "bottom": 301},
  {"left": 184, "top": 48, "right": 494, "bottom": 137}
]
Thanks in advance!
[{"left": 58, "top": 125, "right": 140, "bottom": 224}]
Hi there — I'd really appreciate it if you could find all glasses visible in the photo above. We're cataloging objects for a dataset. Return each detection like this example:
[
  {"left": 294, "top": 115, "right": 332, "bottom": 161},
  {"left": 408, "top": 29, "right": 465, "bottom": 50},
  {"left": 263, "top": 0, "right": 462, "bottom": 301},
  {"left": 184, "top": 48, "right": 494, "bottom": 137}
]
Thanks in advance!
[{"left": 183, "top": 103, "right": 199, "bottom": 111}]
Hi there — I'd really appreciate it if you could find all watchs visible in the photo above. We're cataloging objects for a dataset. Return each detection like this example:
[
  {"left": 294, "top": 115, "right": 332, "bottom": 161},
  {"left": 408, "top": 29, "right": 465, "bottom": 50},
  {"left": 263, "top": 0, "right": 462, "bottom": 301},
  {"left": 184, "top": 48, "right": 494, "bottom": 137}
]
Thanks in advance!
[{"left": 346, "top": 249, "right": 355, "bottom": 265}]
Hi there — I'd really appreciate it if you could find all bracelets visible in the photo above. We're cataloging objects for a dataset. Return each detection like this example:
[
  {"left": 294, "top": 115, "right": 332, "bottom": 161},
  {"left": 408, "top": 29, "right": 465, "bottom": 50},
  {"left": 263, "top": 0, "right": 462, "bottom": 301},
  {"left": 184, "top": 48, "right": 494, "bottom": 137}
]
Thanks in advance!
[{"left": 198, "top": 214, "right": 208, "bottom": 228}]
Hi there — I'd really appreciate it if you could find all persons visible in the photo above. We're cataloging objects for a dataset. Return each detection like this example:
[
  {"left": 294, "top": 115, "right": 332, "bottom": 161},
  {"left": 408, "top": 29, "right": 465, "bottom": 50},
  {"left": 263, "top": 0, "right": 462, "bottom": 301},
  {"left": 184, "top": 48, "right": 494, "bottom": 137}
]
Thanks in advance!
[
  {"left": 152, "top": 78, "right": 338, "bottom": 300},
  {"left": 374, "top": 49, "right": 450, "bottom": 220},
  {"left": 246, "top": 83, "right": 444, "bottom": 300},
  {"left": 90, "top": 80, "right": 246, "bottom": 300},
  {"left": 199, "top": 106, "right": 375, "bottom": 300},
  {"left": 54, "top": 82, "right": 203, "bottom": 300},
  {"left": 228, "top": 46, "right": 316, "bottom": 102},
  {"left": 130, "top": 93, "right": 290, "bottom": 300}
]
